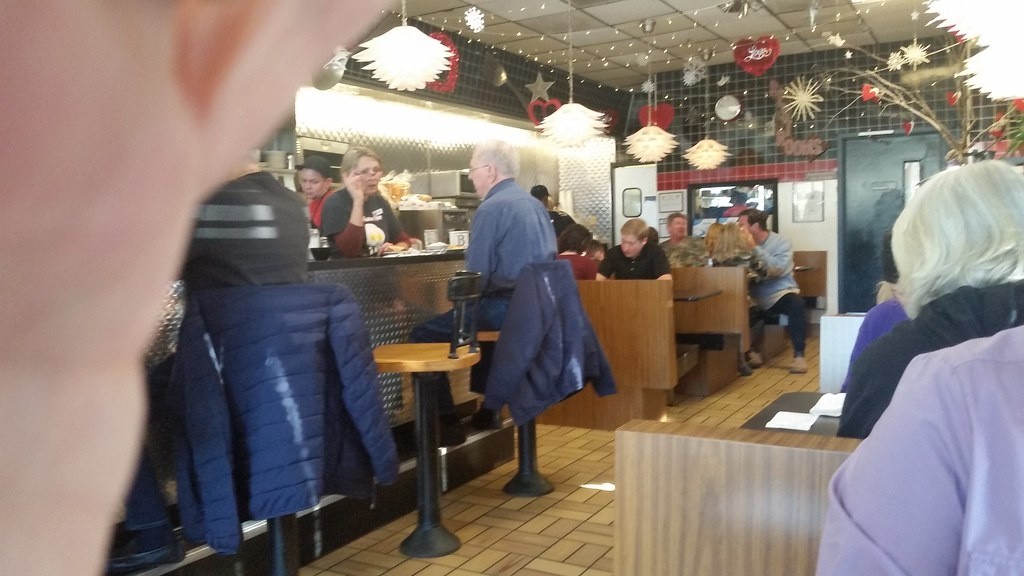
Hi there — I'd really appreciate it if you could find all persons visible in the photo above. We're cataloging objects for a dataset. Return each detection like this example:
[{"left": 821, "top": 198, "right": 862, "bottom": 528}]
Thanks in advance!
[
  {"left": 295, "top": 154, "right": 336, "bottom": 261},
  {"left": 813, "top": 159, "right": 1024, "bottom": 576},
  {"left": 0, "top": 0, "right": 400, "bottom": 576},
  {"left": 105, "top": 151, "right": 310, "bottom": 576},
  {"left": 406, "top": 141, "right": 558, "bottom": 450},
  {"left": 317, "top": 147, "right": 412, "bottom": 260},
  {"left": 532, "top": 183, "right": 810, "bottom": 374}
]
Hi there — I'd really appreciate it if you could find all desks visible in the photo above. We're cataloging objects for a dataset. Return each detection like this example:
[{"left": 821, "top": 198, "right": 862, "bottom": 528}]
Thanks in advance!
[
  {"left": 739, "top": 391, "right": 842, "bottom": 439},
  {"left": 670, "top": 287, "right": 721, "bottom": 302},
  {"left": 788, "top": 264, "right": 814, "bottom": 270}
]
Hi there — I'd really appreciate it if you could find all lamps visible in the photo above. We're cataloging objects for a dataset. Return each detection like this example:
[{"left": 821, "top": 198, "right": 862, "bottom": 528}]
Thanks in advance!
[{"left": 350, "top": 0, "right": 731, "bottom": 170}]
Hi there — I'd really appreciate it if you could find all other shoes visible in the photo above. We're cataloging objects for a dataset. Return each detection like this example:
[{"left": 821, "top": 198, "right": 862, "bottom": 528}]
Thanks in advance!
[
  {"left": 440, "top": 420, "right": 467, "bottom": 446},
  {"left": 789, "top": 359, "right": 810, "bottom": 373},
  {"left": 472, "top": 409, "right": 502, "bottom": 430},
  {"left": 105, "top": 538, "right": 182, "bottom": 576},
  {"left": 747, "top": 351, "right": 763, "bottom": 369}
]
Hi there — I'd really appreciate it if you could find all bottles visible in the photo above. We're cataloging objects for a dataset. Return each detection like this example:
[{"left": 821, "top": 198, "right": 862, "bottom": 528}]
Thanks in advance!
[{"left": 308, "top": 228, "right": 330, "bottom": 261}]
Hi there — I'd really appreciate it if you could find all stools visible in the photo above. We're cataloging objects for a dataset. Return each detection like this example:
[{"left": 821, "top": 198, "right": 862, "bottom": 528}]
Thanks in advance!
[
  {"left": 468, "top": 258, "right": 571, "bottom": 497},
  {"left": 366, "top": 268, "right": 482, "bottom": 559}
]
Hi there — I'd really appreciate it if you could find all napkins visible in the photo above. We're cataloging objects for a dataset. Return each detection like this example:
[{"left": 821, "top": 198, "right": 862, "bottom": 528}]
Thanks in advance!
[
  {"left": 810, "top": 392, "right": 846, "bottom": 416},
  {"left": 764, "top": 411, "right": 818, "bottom": 431}
]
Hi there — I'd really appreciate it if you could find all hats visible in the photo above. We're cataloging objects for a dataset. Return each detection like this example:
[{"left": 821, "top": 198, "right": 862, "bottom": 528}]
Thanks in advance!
[
  {"left": 531, "top": 185, "right": 552, "bottom": 198},
  {"left": 295, "top": 155, "right": 333, "bottom": 178}
]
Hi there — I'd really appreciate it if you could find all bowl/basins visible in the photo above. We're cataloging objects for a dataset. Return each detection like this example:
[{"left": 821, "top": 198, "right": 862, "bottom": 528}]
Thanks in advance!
[{"left": 310, "top": 247, "right": 331, "bottom": 261}]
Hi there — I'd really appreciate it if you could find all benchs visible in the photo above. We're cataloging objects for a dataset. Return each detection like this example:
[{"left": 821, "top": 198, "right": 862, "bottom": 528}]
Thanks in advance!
[{"left": 501, "top": 251, "right": 828, "bottom": 426}]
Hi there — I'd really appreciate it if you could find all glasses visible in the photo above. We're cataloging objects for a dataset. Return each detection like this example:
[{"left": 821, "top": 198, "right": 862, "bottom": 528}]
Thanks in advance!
[
  {"left": 469, "top": 164, "right": 488, "bottom": 175},
  {"left": 355, "top": 168, "right": 379, "bottom": 178}
]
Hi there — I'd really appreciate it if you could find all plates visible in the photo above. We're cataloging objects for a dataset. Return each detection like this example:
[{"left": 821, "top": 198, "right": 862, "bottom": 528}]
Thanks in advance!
[{"left": 424, "top": 245, "right": 448, "bottom": 250}]
